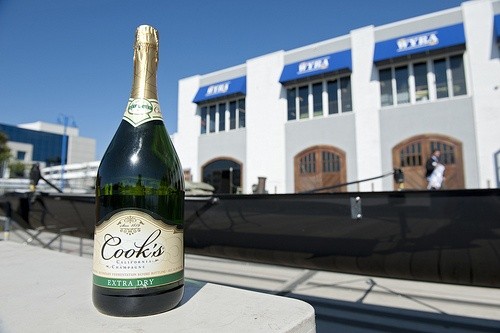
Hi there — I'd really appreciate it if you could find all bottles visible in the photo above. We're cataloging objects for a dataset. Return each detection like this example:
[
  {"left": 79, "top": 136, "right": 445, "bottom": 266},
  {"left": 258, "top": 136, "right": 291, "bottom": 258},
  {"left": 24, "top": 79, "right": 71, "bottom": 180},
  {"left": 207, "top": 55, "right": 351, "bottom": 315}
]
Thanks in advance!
[{"left": 92, "top": 23, "right": 187, "bottom": 318}]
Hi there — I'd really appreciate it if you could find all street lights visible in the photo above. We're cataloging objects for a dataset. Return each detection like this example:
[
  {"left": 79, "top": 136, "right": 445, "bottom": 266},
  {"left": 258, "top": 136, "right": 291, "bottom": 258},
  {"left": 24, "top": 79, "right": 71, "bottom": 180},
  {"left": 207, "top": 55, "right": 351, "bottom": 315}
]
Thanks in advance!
[{"left": 56, "top": 113, "right": 78, "bottom": 195}]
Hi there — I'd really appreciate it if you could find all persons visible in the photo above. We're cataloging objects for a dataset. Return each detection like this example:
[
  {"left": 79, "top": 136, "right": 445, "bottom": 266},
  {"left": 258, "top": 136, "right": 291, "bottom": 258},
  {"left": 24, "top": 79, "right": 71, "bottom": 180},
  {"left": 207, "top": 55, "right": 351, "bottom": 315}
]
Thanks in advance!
[
  {"left": 425, "top": 149, "right": 446, "bottom": 190},
  {"left": 28, "top": 163, "right": 42, "bottom": 189}
]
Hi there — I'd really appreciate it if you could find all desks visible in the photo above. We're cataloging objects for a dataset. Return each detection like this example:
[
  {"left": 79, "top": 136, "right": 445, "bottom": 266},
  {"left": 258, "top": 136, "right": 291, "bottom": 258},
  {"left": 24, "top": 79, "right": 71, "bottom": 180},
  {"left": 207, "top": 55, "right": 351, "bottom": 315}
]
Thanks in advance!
[{"left": 0, "top": 239, "right": 319, "bottom": 333}]
